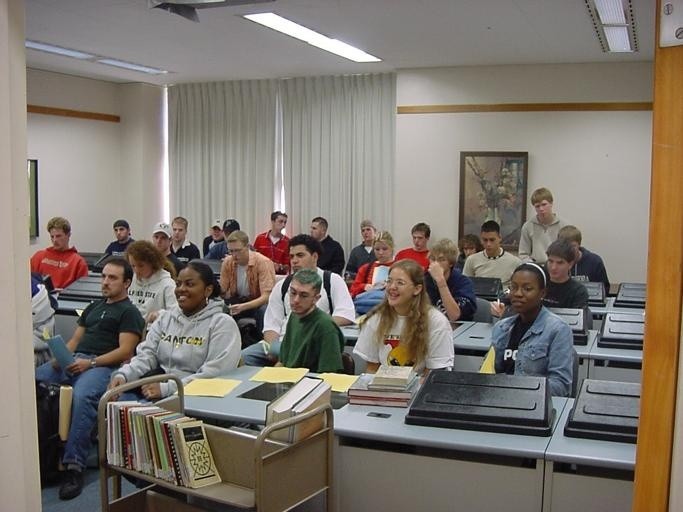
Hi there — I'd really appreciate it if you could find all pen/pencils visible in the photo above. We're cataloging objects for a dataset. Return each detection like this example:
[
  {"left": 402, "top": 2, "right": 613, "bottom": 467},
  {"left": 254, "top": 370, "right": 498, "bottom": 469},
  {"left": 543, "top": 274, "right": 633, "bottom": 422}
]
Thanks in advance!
[
  {"left": 496, "top": 294, "right": 500, "bottom": 310},
  {"left": 229, "top": 303, "right": 233, "bottom": 317}
]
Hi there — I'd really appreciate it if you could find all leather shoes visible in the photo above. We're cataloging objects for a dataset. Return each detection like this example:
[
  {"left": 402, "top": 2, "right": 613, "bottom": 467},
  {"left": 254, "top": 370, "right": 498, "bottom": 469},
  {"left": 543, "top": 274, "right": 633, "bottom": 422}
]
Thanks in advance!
[{"left": 58, "top": 467, "right": 85, "bottom": 501}]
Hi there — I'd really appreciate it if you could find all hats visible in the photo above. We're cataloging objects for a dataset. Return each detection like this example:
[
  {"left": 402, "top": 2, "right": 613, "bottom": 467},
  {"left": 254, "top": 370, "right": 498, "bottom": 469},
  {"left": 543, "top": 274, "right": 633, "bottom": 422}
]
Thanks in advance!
[
  {"left": 210, "top": 218, "right": 224, "bottom": 231},
  {"left": 153, "top": 222, "right": 173, "bottom": 239},
  {"left": 360, "top": 219, "right": 377, "bottom": 232},
  {"left": 223, "top": 218, "right": 240, "bottom": 233}
]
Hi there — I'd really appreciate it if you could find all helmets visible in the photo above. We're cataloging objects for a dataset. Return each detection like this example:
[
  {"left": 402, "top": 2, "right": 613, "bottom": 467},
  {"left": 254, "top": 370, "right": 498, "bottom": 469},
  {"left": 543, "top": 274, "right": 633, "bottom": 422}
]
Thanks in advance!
[{"left": 113, "top": 219, "right": 131, "bottom": 236}]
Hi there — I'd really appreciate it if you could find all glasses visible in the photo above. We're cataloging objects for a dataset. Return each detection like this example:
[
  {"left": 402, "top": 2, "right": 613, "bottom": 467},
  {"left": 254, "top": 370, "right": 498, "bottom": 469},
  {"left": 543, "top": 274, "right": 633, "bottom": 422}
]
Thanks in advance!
[
  {"left": 384, "top": 279, "right": 415, "bottom": 288},
  {"left": 507, "top": 285, "right": 536, "bottom": 294}
]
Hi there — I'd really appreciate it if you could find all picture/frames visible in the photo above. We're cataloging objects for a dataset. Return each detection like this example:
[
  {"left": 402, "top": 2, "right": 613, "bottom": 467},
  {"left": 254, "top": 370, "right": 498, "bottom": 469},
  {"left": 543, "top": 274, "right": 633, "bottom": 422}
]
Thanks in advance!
[
  {"left": 458, "top": 151, "right": 528, "bottom": 250},
  {"left": 27, "top": 159, "right": 39, "bottom": 237}
]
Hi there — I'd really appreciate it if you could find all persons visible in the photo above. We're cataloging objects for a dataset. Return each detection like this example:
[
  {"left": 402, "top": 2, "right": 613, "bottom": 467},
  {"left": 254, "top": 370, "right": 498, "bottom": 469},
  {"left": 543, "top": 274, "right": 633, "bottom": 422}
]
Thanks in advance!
[
  {"left": 558, "top": 225, "right": 609, "bottom": 296},
  {"left": 490, "top": 238, "right": 588, "bottom": 318},
  {"left": 461, "top": 221, "right": 519, "bottom": 295},
  {"left": 479, "top": 262, "right": 574, "bottom": 396},
  {"left": 519, "top": 188, "right": 567, "bottom": 265},
  {"left": 31, "top": 210, "right": 479, "bottom": 501}
]
